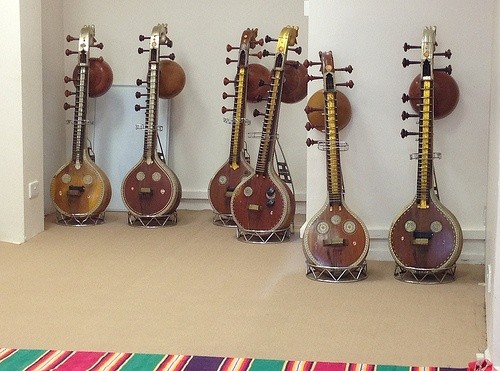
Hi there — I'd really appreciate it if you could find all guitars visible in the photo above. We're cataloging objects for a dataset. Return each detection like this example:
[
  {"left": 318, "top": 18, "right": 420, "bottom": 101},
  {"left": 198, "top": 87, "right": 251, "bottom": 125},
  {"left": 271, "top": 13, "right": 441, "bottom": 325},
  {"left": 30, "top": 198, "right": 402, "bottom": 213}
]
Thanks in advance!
[
  {"left": 206, "top": 27, "right": 271, "bottom": 228},
  {"left": 230, "top": 24, "right": 308, "bottom": 244},
  {"left": 119, "top": 22, "right": 186, "bottom": 229},
  {"left": 304, "top": 50, "right": 370, "bottom": 282},
  {"left": 388, "top": 24, "right": 464, "bottom": 283},
  {"left": 50, "top": 24, "right": 114, "bottom": 227}
]
